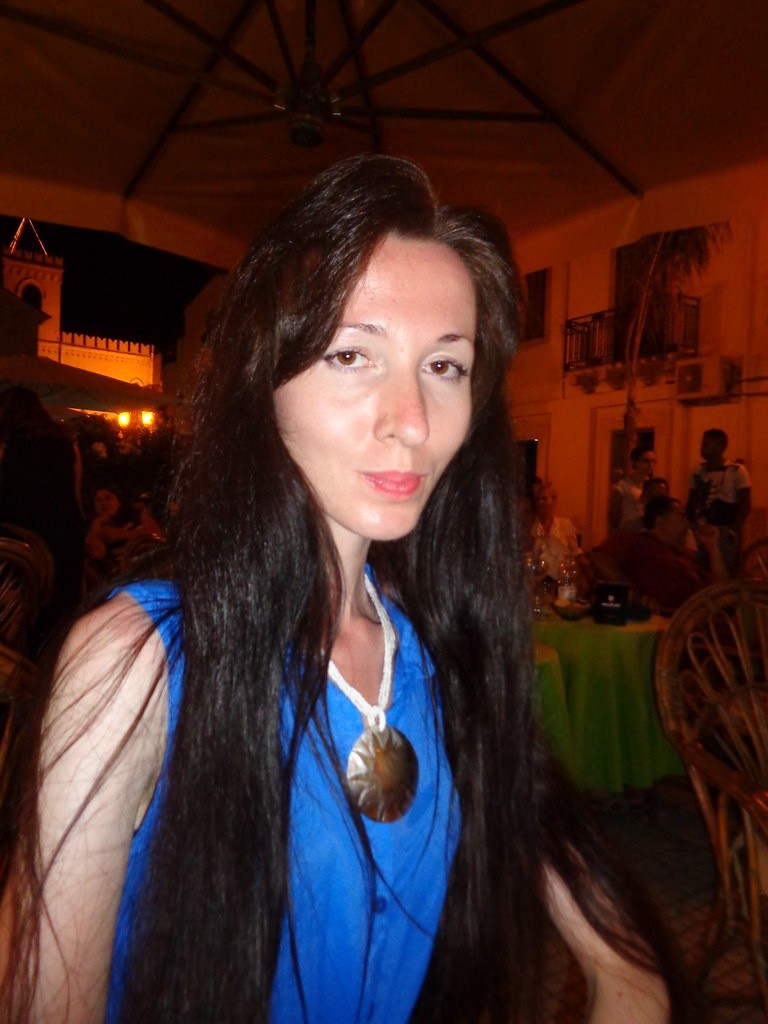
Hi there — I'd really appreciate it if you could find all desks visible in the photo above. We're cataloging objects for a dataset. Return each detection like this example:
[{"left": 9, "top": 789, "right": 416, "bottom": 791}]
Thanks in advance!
[{"left": 532, "top": 607, "right": 685, "bottom": 792}]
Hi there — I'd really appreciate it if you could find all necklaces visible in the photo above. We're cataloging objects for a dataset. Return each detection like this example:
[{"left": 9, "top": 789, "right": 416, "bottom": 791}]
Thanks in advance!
[{"left": 330, "top": 576, "right": 419, "bottom": 823}]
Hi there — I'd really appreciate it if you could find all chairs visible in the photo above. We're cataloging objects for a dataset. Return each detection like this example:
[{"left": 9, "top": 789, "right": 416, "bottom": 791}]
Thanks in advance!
[
  {"left": 0, "top": 525, "right": 56, "bottom": 869},
  {"left": 656, "top": 536, "right": 768, "bottom": 1024}
]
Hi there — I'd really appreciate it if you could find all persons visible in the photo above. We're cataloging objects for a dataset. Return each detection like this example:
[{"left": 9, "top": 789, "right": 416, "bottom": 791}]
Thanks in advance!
[{"left": 0, "top": 151, "right": 751, "bottom": 1024}]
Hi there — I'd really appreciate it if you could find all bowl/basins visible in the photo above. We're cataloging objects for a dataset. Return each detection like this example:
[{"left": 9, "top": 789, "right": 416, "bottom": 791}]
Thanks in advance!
[{"left": 552, "top": 599, "right": 591, "bottom": 620}]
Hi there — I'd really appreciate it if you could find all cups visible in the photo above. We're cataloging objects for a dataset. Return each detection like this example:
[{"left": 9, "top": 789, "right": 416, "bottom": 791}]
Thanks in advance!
[{"left": 533, "top": 582, "right": 554, "bottom": 620}]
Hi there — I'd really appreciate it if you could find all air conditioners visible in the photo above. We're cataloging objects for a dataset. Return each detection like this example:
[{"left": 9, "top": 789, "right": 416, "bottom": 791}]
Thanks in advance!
[{"left": 676, "top": 356, "right": 728, "bottom": 400}]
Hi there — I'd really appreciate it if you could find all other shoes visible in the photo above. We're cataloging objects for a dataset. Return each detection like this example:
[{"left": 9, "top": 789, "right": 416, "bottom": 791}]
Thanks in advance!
[{"left": 683, "top": 723, "right": 713, "bottom": 742}]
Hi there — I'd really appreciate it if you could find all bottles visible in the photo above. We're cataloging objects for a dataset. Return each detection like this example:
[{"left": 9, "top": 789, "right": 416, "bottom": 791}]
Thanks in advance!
[{"left": 558, "top": 553, "right": 579, "bottom": 600}]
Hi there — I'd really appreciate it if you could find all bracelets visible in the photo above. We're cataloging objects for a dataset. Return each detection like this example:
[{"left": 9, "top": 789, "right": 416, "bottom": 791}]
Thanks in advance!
[{"left": 729, "top": 530, "right": 737, "bottom": 537}]
